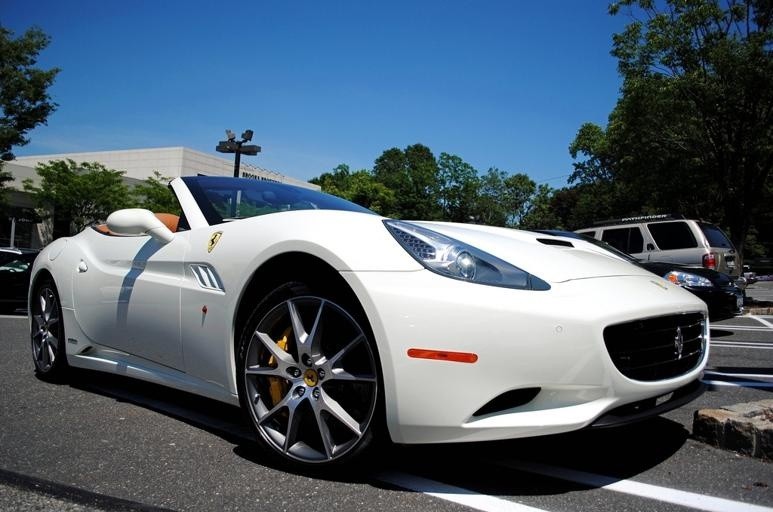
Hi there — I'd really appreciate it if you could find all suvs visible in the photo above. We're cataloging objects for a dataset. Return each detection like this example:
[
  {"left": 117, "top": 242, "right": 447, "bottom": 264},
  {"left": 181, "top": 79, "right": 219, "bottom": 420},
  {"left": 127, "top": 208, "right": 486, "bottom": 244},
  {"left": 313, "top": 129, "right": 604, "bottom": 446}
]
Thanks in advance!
[
  {"left": 1, "top": 246, "right": 43, "bottom": 271},
  {"left": 566, "top": 211, "right": 747, "bottom": 291}
]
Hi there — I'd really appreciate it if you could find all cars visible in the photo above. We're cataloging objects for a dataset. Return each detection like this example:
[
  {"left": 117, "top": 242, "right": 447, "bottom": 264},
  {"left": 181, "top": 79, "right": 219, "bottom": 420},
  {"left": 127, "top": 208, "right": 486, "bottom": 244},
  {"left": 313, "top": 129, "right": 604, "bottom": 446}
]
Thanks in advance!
[
  {"left": 1, "top": 252, "right": 38, "bottom": 313},
  {"left": 743, "top": 264, "right": 758, "bottom": 282},
  {"left": 533, "top": 227, "right": 744, "bottom": 322}
]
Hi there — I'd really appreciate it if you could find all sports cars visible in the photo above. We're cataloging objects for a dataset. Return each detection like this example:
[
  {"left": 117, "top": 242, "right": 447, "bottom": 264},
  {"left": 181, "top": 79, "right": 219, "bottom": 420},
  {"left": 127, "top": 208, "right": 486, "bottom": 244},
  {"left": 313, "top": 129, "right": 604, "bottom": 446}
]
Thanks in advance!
[{"left": 15, "top": 172, "right": 714, "bottom": 480}]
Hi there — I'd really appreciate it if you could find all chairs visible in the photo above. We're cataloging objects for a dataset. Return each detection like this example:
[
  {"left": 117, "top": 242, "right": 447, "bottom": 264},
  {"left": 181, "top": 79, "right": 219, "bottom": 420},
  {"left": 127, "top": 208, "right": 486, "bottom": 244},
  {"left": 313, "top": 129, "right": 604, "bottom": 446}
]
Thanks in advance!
[{"left": 97, "top": 211, "right": 180, "bottom": 235}]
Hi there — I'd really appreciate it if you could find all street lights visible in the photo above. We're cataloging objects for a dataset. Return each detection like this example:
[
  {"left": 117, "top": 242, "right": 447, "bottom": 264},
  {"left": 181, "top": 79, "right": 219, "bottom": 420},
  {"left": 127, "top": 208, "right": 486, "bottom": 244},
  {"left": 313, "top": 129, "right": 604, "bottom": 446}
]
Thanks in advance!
[{"left": 214, "top": 125, "right": 263, "bottom": 217}]
